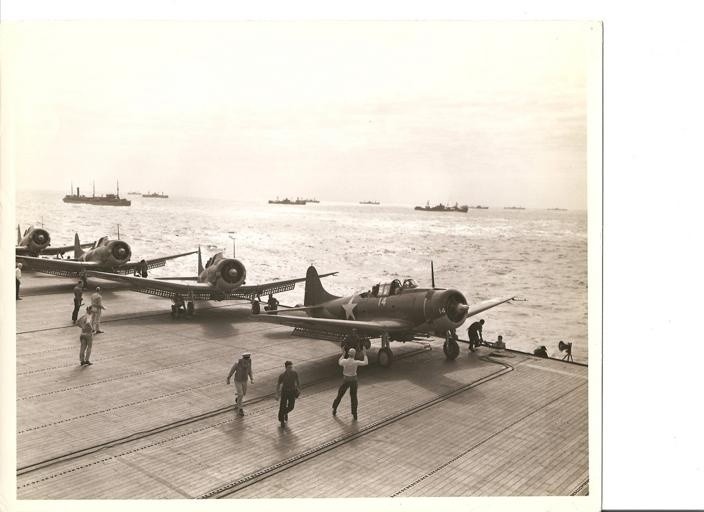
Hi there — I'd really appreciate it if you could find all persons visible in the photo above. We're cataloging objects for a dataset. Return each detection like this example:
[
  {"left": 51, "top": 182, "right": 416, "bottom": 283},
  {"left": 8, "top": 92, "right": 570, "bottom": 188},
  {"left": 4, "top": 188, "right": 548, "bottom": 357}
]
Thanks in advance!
[
  {"left": 15, "top": 263, "right": 23, "bottom": 300},
  {"left": 169, "top": 299, "right": 185, "bottom": 315},
  {"left": 90, "top": 286, "right": 105, "bottom": 333},
  {"left": 466, "top": 319, "right": 484, "bottom": 349},
  {"left": 132, "top": 259, "right": 146, "bottom": 276},
  {"left": 330, "top": 346, "right": 369, "bottom": 420},
  {"left": 275, "top": 360, "right": 300, "bottom": 428},
  {"left": 75, "top": 305, "right": 93, "bottom": 366},
  {"left": 225, "top": 352, "right": 255, "bottom": 415},
  {"left": 263, "top": 293, "right": 278, "bottom": 314},
  {"left": 55, "top": 249, "right": 64, "bottom": 259},
  {"left": 533, "top": 345, "right": 549, "bottom": 357},
  {"left": 491, "top": 336, "right": 506, "bottom": 348},
  {"left": 71, "top": 281, "right": 83, "bottom": 323}
]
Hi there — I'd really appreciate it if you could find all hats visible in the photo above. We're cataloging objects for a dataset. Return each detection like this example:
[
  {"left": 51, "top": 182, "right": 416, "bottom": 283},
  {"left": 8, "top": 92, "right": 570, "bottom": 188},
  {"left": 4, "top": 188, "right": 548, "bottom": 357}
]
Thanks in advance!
[{"left": 242, "top": 353, "right": 251, "bottom": 359}]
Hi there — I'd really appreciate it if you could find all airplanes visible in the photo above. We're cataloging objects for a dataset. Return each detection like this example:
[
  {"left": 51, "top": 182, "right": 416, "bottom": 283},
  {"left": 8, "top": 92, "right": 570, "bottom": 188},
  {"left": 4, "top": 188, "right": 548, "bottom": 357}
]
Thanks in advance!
[{"left": 248, "top": 266, "right": 528, "bottom": 369}]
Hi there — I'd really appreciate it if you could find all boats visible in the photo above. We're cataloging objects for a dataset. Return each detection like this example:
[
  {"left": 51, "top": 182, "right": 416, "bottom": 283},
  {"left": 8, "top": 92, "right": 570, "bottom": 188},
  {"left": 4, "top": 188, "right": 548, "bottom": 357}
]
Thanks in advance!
[
  {"left": 504, "top": 205, "right": 525, "bottom": 209},
  {"left": 414, "top": 200, "right": 488, "bottom": 212},
  {"left": 129, "top": 192, "right": 168, "bottom": 198},
  {"left": 360, "top": 201, "right": 380, "bottom": 204},
  {"left": 269, "top": 196, "right": 320, "bottom": 205},
  {"left": 62, "top": 179, "right": 131, "bottom": 206},
  {"left": 547, "top": 208, "right": 568, "bottom": 210}
]
